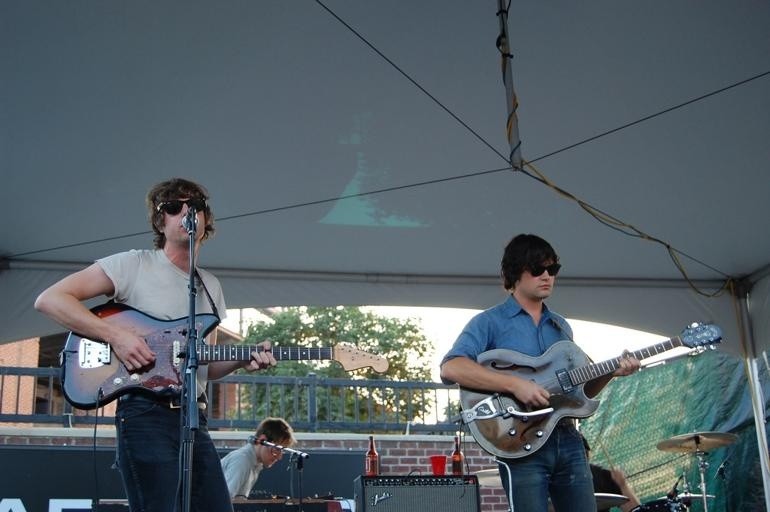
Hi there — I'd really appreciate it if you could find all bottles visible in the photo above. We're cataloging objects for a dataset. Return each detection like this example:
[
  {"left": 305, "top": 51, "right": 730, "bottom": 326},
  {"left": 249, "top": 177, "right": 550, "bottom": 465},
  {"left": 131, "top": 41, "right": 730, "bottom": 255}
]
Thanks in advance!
[
  {"left": 451, "top": 436, "right": 464, "bottom": 475},
  {"left": 365, "top": 435, "right": 379, "bottom": 476}
]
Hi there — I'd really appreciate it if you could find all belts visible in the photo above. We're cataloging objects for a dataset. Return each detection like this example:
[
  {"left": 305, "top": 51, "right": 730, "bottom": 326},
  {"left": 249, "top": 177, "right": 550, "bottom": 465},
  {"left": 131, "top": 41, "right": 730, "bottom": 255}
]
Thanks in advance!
[{"left": 119, "top": 389, "right": 207, "bottom": 411}]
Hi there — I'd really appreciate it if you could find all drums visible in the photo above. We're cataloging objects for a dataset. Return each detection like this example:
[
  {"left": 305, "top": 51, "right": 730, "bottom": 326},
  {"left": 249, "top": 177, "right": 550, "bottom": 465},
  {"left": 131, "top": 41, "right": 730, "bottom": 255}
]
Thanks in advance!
[{"left": 629, "top": 499, "right": 688, "bottom": 512}]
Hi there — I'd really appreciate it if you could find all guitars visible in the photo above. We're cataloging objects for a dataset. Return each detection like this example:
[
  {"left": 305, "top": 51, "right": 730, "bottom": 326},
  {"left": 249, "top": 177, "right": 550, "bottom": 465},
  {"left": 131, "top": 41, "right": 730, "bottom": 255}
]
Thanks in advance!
[
  {"left": 59, "top": 303, "right": 390, "bottom": 409},
  {"left": 458, "top": 323, "right": 722, "bottom": 460}
]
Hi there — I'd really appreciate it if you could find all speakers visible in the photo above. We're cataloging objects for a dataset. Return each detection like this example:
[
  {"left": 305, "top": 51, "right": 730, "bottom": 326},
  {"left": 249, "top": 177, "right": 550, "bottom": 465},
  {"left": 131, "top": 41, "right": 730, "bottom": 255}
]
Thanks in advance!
[{"left": 353, "top": 473, "right": 481, "bottom": 512}]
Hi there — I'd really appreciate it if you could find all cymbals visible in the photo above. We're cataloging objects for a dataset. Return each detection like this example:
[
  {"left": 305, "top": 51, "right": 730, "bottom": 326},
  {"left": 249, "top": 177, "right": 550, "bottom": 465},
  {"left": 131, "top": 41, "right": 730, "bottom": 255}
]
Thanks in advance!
[
  {"left": 548, "top": 493, "right": 629, "bottom": 512},
  {"left": 657, "top": 431, "right": 740, "bottom": 453},
  {"left": 657, "top": 492, "right": 715, "bottom": 502}
]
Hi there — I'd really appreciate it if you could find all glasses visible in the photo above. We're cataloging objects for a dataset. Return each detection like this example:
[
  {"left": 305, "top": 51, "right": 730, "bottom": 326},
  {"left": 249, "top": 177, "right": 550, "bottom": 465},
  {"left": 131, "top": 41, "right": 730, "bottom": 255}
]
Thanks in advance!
[
  {"left": 156, "top": 197, "right": 207, "bottom": 215},
  {"left": 531, "top": 263, "right": 561, "bottom": 277}
]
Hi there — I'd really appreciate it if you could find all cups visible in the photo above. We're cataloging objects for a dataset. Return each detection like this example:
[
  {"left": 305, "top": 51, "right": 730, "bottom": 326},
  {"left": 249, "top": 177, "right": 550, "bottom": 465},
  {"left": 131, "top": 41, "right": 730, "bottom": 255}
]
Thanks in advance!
[{"left": 430, "top": 456, "right": 447, "bottom": 475}]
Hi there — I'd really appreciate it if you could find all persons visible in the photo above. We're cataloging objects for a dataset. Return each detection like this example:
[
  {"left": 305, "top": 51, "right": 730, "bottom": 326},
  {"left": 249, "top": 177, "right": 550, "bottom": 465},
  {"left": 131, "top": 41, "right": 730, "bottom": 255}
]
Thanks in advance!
[
  {"left": 218, "top": 415, "right": 298, "bottom": 504},
  {"left": 437, "top": 231, "right": 643, "bottom": 511},
  {"left": 578, "top": 433, "right": 642, "bottom": 511},
  {"left": 33, "top": 179, "right": 279, "bottom": 511}
]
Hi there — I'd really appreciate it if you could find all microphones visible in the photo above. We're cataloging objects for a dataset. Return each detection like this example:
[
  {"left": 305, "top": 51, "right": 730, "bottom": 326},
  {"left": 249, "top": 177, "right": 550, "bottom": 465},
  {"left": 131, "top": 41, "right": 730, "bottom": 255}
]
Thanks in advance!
[
  {"left": 248, "top": 435, "right": 268, "bottom": 448},
  {"left": 182, "top": 206, "right": 200, "bottom": 232}
]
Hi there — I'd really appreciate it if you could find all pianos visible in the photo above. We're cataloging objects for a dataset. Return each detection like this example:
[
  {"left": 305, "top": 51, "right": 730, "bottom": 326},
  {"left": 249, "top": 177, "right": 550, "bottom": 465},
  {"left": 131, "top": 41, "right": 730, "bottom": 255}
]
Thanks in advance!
[{"left": 232, "top": 496, "right": 355, "bottom": 512}]
